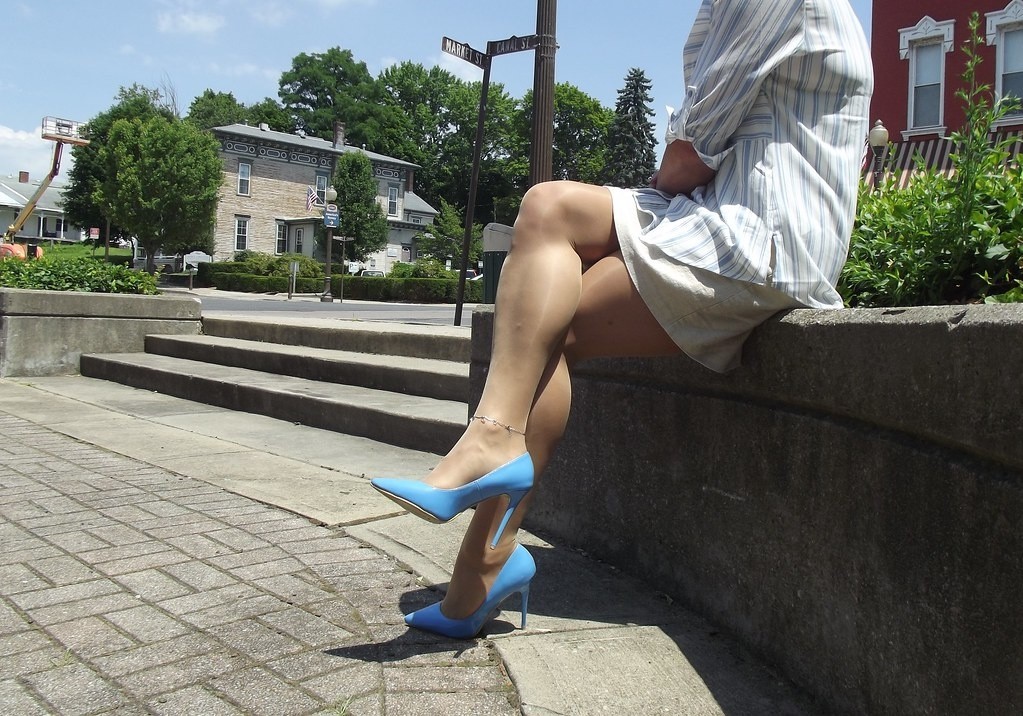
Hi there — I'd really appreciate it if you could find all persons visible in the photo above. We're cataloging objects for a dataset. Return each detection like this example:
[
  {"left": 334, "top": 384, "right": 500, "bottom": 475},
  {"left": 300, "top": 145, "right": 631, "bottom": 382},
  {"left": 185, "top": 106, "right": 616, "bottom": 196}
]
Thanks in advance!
[{"left": 368, "top": 0, "right": 877, "bottom": 644}]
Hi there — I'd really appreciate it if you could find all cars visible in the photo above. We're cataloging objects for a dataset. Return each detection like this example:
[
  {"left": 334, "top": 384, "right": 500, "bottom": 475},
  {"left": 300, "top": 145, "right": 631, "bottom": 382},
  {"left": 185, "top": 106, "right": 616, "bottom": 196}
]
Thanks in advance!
[{"left": 359, "top": 270, "right": 386, "bottom": 279}]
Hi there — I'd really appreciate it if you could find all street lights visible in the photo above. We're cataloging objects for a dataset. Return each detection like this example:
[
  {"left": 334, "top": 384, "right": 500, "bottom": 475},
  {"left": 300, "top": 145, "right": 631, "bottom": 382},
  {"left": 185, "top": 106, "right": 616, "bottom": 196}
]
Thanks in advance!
[
  {"left": 870, "top": 120, "right": 889, "bottom": 192},
  {"left": 321, "top": 182, "right": 338, "bottom": 303}
]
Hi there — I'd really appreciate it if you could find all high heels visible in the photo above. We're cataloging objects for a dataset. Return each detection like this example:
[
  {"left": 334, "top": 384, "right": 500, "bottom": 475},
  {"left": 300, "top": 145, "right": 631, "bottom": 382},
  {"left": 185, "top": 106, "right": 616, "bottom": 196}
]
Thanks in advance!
[
  {"left": 404, "top": 543, "right": 536, "bottom": 639},
  {"left": 370, "top": 451, "right": 534, "bottom": 550}
]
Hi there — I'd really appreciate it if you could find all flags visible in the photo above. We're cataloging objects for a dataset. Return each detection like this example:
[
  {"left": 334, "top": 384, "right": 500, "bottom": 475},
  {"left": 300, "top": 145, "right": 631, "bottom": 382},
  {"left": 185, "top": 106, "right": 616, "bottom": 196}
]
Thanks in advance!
[{"left": 305, "top": 185, "right": 318, "bottom": 213}]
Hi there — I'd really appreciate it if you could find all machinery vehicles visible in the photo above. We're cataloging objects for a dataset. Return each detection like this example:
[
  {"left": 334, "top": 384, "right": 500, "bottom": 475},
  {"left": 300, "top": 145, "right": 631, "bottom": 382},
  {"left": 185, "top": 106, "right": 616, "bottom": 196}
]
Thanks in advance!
[{"left": 0, "top": 115, "right": 91, "bottom": 261}]
